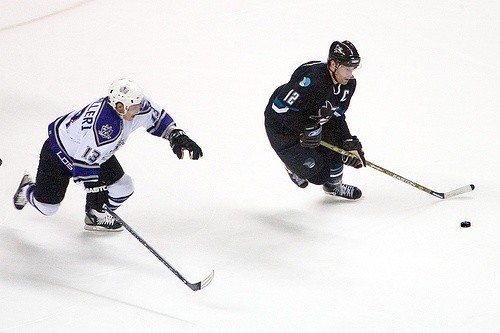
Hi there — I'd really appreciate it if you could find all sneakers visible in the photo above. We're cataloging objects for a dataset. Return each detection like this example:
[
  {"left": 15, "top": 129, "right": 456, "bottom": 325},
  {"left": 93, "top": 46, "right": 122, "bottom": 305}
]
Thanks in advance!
[
  {"left": 286, "top": 167, "right": 308, "bottom": 189},
  {"left": 84, "top": 209, "right": 123, "bottom": 231},
  {"left": 322, "top": 182, "right": 362, "bottom": 200},
  {"left": 14, "top": 174, "right": 33, "bottom": 209}
]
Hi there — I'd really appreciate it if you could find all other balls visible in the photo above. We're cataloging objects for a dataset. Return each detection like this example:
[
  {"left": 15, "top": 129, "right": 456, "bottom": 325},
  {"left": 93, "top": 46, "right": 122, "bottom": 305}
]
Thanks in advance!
[{"left": 459, "top": 221, "right": 472, "bottom": 228}]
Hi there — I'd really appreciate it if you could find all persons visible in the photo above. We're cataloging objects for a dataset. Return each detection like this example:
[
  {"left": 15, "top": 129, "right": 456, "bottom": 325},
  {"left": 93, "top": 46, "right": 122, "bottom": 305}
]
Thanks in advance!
[
  {"left": 263, "top": 40, "right": 366, "bottom": 199},
  {"left": 14, "top": 80, "right": 203, "bottom": 232}
]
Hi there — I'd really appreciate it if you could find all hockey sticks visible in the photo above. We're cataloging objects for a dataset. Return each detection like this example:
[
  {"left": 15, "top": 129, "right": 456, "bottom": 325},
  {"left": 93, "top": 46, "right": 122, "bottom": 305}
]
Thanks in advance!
[
  {"left": 320, "top": 141, "right": 475, "bottom": 200},
  {"left": 102, "top": 202, "right": 214, "bottom": 292}
]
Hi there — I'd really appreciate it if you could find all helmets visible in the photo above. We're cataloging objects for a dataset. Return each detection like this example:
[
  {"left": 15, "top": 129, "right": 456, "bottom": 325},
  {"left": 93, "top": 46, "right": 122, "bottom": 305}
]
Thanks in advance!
[
  {"left": 330, "top": 41, "right": 360, "bottom": 67},
  {"left": 108, "top": 78, "right": 143, "bottom": 114}
]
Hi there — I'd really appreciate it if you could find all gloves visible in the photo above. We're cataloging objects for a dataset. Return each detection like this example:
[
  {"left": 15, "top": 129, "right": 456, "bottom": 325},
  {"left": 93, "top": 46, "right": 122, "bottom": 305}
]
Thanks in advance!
[
  {"left": 83, "top": 181, "right": 110, "bottom": 213},
  {"left": 299, "top": 119, "right": 322, "bottom": 149},
  {"left": 340, "top": 137, "right": 366, "bottom": 169},
  {"left": 168, "top": 129, "right": 203, "bottom": 160}
]
